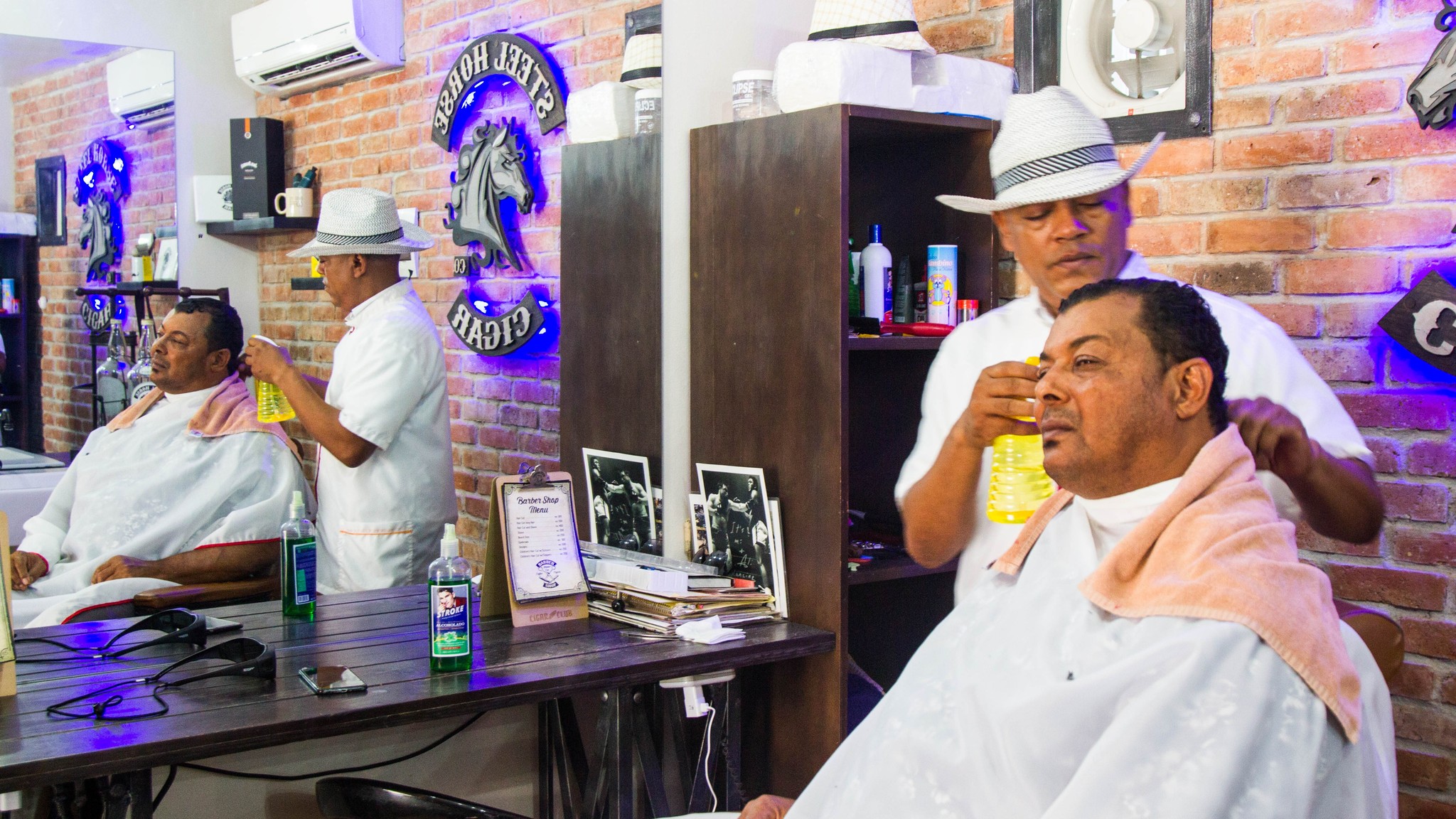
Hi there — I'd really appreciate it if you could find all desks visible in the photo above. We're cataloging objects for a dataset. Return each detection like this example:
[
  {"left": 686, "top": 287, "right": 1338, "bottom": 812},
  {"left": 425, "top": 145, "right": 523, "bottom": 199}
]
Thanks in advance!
[
  {"left": 0, "top": 619, "right": 836, "bottom": 819},
  {"left": 12, "top": 579, "right": 480, "bottom": 683}
]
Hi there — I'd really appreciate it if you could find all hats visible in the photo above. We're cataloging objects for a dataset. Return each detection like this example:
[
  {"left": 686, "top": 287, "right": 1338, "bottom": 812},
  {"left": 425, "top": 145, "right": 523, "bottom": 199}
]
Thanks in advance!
[
  {"left": 935, "top": 85, "right": 1165, "bottom": 216},
  {"left": 286, "top": 187, "right": 435, "bottom": 258},
  {"left": 807, "top": 2, "right": 936, "bottom": 52},
  {"left": 619, "top": 34, "right": 663, "bottom": 91}
]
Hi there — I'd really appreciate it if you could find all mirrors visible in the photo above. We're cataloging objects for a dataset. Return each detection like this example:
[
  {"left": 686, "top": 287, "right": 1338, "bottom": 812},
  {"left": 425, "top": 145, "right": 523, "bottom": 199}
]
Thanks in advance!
[
  {"left": 0, "top": 32, "right": 179, "bottom": 475},
  {"left": 1, "top": 0, "right": 662, "bottom": 689},
  {"left": 34, "top": 156, "right": 68, "bottom": 247}
]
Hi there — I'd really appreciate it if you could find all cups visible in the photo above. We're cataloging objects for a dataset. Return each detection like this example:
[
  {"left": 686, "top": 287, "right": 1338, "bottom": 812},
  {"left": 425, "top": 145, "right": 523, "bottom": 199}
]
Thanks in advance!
[{"left": 275, "top": 187, "right": 314, "bottom": 217}]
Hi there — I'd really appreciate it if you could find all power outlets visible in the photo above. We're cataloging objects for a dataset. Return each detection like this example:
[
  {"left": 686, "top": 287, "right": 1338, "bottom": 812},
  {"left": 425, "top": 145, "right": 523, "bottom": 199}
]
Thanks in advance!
[{"left": 659, "top": 668, "right": 736, "bottom": 688}]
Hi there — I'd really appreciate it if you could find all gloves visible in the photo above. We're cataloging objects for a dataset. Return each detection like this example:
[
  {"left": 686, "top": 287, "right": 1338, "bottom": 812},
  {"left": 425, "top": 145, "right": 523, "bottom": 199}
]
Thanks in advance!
[
  {"left": 626, "top": 489, "right": 638, "bottom": 502},
  {"left": 716, "top": 500, "right": 728, "bottom": 515},
  {"left": 733, "top": 497, "right": 741, "bottom": 504},
  {"left": 597, "top": 477, "right": 606, "bottom": 488}
]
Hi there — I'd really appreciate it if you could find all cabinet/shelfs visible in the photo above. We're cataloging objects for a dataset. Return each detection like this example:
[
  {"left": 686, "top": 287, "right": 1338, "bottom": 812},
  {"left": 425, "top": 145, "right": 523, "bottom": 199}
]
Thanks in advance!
[
  {"left": 206, "top": 216, "right": 326, "bottom": 291},
  {"left": 690, "top": 103, "right": 1002, "bottom": 814},
  {"left": 561, "top": 134, "right": 662, "bottom": 545}
]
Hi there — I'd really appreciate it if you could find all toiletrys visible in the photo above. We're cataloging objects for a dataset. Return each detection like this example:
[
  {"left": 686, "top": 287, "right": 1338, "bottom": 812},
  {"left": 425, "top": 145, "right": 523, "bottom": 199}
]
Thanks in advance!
[
  {"left": 427, "top": 523, "right": 473, "bottom": 673},
  {"left": 252, "top": 335, "right": 297, "bottom": 423},
  {"left": 986, "top": 354, "right": 1055, "bottom": 523},
  {"left": 847, "top": 238, "right": 860, "bottom": 331},
  {"left": 894, "top": 251, "right": 915, "bottom": 335},
  {"left": 860, "top": 223, "right": 893, "bottom": 336},
  {"left": 280, "top": 491, "right": 320, "bottom": 618}
]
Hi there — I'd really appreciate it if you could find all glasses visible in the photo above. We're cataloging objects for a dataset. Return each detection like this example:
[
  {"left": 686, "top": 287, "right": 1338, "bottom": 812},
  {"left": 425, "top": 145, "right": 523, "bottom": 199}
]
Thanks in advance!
[
  {"left": 97, "top": 608, "right": 207, "bottom": 657},
  {"left": 147, "top": 636, "right": 277, "bottom": 686}
]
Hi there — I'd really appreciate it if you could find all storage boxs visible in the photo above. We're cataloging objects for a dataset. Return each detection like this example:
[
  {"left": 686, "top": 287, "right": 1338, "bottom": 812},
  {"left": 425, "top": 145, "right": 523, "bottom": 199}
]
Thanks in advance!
[{"left": 229, "top": 118, "right": 284, "bottom": 221}]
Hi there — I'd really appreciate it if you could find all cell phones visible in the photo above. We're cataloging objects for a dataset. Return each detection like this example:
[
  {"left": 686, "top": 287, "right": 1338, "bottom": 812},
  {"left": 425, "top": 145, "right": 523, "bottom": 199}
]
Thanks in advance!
[
  {"left": 299, "top": 665, "right": 366, "bottom": 695},
  {"left": 205, "top": 615, "right": 244, "bottom": 634}
]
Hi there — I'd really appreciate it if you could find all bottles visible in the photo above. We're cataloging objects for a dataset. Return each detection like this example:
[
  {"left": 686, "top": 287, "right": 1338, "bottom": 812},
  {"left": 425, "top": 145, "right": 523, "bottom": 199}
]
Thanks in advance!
[
  {"left": 96, "top": 319, "right": 135, "bottom": 427},
  {"left": 2, "top": 278, "right": 14, "bottom": 314},
  {"left": 293, "top": 173, "right": 302, "bottom": 188},
  {"left": 298, "top": 166, "right": 318, "bottom": 187},
  {"left": 731, "top": 69, "right": 781, "bottom": 121},
  {"left": 858, "top": 224, "right": 892, "bottom": 337},
  {"left": 127, "top": 319, "right": 158, "bottom": 405},
  {"left": 635, "top": 90, "right": 663, "bottom": 136},
  {"left": 927, "top": 245, "right": 957, "bottom": 327},
  {"left": 957, "top": 299, "right": 978, "bottom": 324},
  {"left": 12, "top": 299, "right": 20, "bottom": 314}
]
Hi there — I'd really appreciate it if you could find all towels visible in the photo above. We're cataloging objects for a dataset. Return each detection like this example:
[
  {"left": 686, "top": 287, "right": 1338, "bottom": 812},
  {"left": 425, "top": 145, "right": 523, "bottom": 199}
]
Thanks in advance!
[
  {"left": 989, "top": 422, "right": 1364, "bottom": 744},
  {"left": 105, "top": 369, "right": 303, "bottom": 472}
]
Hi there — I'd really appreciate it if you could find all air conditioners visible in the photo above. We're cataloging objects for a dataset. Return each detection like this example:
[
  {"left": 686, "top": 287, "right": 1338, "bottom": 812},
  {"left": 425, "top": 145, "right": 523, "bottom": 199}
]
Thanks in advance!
[
  {"left": 231, "top": 0, "right": 406, "bottom": 99},
  {"left": 107, "top": 47, "right": 175, "bottom": 130}
]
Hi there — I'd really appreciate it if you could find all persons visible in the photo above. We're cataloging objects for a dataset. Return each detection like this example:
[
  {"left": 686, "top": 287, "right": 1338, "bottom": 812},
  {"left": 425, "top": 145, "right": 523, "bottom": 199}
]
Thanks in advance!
[
  {"left": 0, "top": 298, "right": 318, "bottom": 629},
  {"left": 735, "top": 278, "right": 1402, "bottom": 819},
  {"left": 893, "top": 84, "right": 1385, "bottom": 609},
  {"left": 239, "top": 187, "right": 459, "bottom": 593},
  {"left": 592, "top": 459, "right": 772, "bottom": 594}
]
